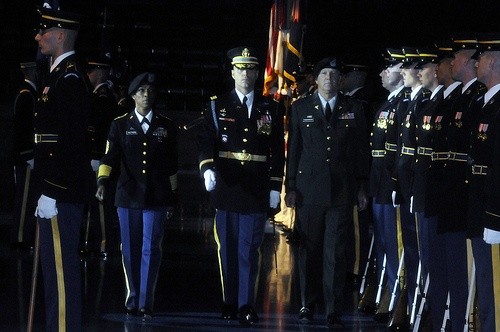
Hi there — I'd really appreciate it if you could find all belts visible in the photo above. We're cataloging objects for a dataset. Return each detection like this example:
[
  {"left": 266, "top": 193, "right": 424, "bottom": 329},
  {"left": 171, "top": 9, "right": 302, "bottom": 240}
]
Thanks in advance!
[
  {"left": 218, "top": 151, "right": 267, "bottom": 162},
  {"left": 372, "top": 141, "right": 488, "bottom": 175},
  {"left": 35, "top": 134, "right": 57, "bottom": 142}
]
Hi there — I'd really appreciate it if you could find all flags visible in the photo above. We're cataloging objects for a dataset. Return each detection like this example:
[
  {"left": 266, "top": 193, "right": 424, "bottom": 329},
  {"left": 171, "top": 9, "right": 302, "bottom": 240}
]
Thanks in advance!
[{"left": 261, "top": 0, "right": 304, "bottom": 100}]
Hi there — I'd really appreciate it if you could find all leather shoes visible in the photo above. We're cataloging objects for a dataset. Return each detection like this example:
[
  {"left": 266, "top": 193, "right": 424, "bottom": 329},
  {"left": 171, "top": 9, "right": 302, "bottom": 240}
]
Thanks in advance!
[
  {"left": 299, "top": 306, "right": 314, "bottom": 322},
  {"left": 239, "top": 307, "right": 259, "bottom": 325},
  {"left": 221, "top": 304, "right": 238, "bottom": 320},
  {"left": 327, "top": 314, "right": 345, "bottom": 329}
]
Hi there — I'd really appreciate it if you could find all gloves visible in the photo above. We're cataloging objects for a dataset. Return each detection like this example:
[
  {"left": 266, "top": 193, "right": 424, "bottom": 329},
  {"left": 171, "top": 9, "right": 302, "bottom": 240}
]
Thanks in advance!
[
  {"left": 203, "top": 169, "right": 217, "bottom": 191},
  {"left": 34, "top": 194, "right": 58, "bottom": 219},
  {"left": 269, "top": 190, "right": 280, "bottom": 208}
]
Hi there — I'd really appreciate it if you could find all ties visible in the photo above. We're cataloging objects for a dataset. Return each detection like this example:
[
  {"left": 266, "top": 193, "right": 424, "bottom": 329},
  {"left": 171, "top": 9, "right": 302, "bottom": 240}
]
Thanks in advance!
[
  {"left": 325, "top": 100, "right": 331, "bottom": 122},
  {"left": 242, "top": 96, "right": 248, "bottom": 120},
  {"left": 141, "top": 118, "right": 150, "bottom": 134}
]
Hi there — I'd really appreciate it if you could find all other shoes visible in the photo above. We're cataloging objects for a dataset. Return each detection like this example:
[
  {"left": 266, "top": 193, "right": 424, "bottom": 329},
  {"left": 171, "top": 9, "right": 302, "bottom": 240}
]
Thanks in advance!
[
  {"left": 125, "top": 307, "right": 138, "bottom": 315},
  {"left": 140, "top": 310, "right": 152, "bottom": 318}
]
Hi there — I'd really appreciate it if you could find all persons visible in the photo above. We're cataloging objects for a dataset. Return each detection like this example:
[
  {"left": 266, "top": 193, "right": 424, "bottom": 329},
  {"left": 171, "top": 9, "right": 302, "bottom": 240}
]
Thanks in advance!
[
  {"left": 11, "top": 44, "right": 130, "bottom": 267},
  {"left": 195, "top": 46, "right": 285, "bottom": 326},
  {"left": 338, "top": 35, "right": 500, "bottom": 332},
  {"left": 22, "top": 6, "right": 98, "bottom": 332},
  {"left": 269, "top": 63, "right": 317, "bottom": 244},
  {"left": 94, "top": 70, "right": 179, "bottom": 318},
  {"left": 284, "top": 59, "right": 370, "bottom": 328}
]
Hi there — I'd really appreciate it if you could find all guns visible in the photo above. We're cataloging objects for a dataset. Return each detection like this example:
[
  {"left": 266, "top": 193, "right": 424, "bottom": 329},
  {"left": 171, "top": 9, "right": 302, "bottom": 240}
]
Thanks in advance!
[
  {"left": 386, "top": 281, "right": 408, "bottom": 331},
  {"left": 468, "top": 295, "right": 481, "bottom": 332},
  {"left": 357, "top": 255, "right": 378, "bottom": 313},
  {"left": 373, "top": 275, "right": 391, "bottom": 323}
]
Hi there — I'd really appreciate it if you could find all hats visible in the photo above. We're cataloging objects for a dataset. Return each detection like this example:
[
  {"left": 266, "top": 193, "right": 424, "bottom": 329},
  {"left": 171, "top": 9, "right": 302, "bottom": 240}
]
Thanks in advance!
[
  {"left": 87, "top": 56, "right": 113, "bottom": 70},
  {"left": 314, "top": 57, "right": 343, "bottom": 75},
  {"left": 37, "top": 0, "right": 81, "bottom": 30},
  {"left": 128, "top": 72, "right": 157, "bottom": 95},
  {"left": 341, "top": 63, "right": 370, "bottom": 74},
  {"left": 379, "top": 34, "right": 500, "bottom": 70},
  {"left": 20, "top": 56, "right": 37, "bottom": 68},
  {"left": 226, "top": 46, "right": 261, "bottom": 70}
]
